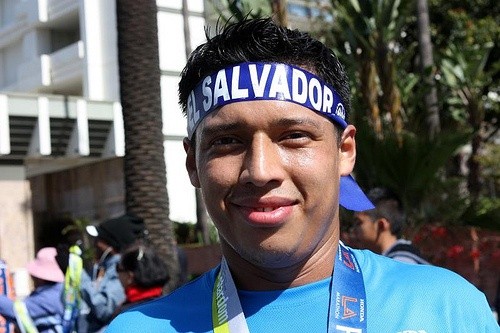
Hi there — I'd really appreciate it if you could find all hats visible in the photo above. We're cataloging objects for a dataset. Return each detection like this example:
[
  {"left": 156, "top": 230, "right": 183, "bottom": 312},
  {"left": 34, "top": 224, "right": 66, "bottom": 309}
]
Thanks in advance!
[
  {"left": 97, "top": 212, "right": 145, "bottom": 252},
  {"left": 27, "top": 247, "right": 65, "bottom": 282}
]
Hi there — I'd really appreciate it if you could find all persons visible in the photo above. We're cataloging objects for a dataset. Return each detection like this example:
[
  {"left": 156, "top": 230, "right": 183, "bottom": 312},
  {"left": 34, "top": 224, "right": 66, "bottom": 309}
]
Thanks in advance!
[
  {"left": 352, "top": 188, "right": 435, "bottom": 266},
  {"left": 96, "top": 7, "right": 500, "bottom": 333},
  {"left": 0, "top": 214, "right": 170, "bottom": 333}
]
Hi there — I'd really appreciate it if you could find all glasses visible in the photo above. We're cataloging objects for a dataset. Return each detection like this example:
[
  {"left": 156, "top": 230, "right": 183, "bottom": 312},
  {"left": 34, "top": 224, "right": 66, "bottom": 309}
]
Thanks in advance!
[{"left": 116, "top": 263, "right": 134, "bottom": 272}]
